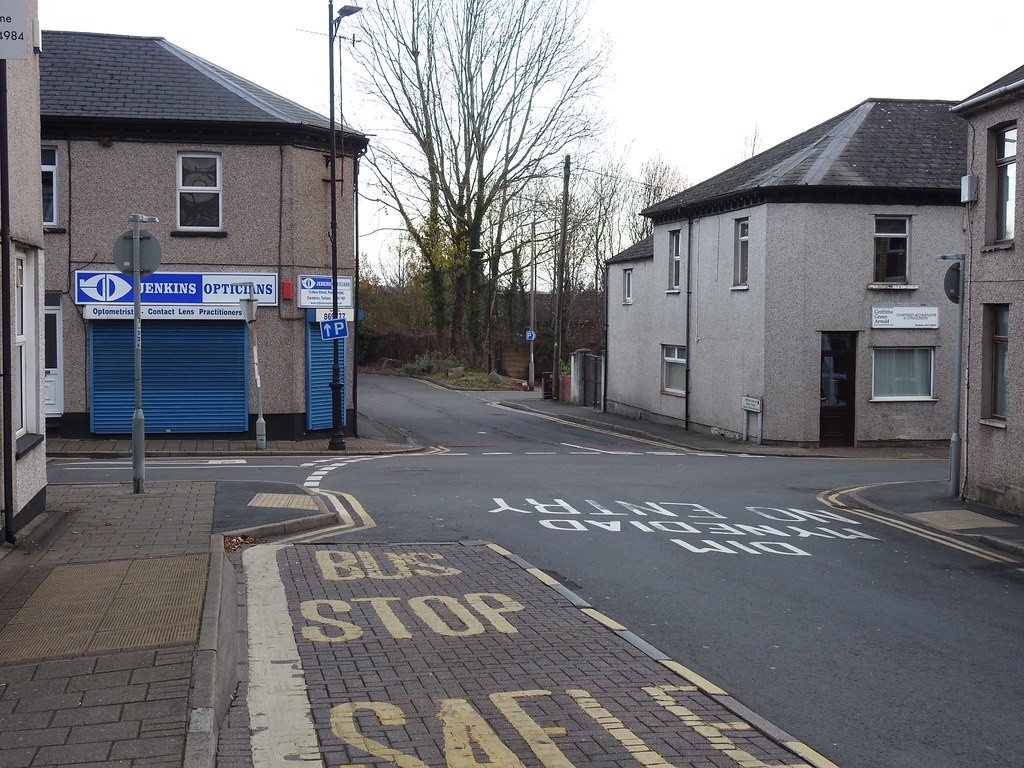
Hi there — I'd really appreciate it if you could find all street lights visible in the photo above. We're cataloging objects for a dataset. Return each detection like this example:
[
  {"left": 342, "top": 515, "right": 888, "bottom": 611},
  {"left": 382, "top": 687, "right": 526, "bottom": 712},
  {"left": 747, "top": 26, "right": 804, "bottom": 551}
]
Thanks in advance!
[
  {"left": 127, "top": 212, "right": 158, "bottom": 492},
  {"left": 938, "top": 254, "right": 965, "bottom": 495},
  {"left": 230, "top": 282, "right": 267, "bottom": 448},
  {"left": 330, "top": 5, "right": 365, "bottom": 448},
  {"left": 472, "top": 249, "right": 492, "bottom": 375},
  {"left": 520, "top": 221, "right": 536, "bottom": 392}
]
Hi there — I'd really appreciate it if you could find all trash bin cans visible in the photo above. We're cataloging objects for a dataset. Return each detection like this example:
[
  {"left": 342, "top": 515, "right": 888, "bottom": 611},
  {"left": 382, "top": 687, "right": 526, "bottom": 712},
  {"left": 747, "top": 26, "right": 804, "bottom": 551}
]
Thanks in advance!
[{"left": 542, "top": 372, "right": 553, "bottom": 400}]
